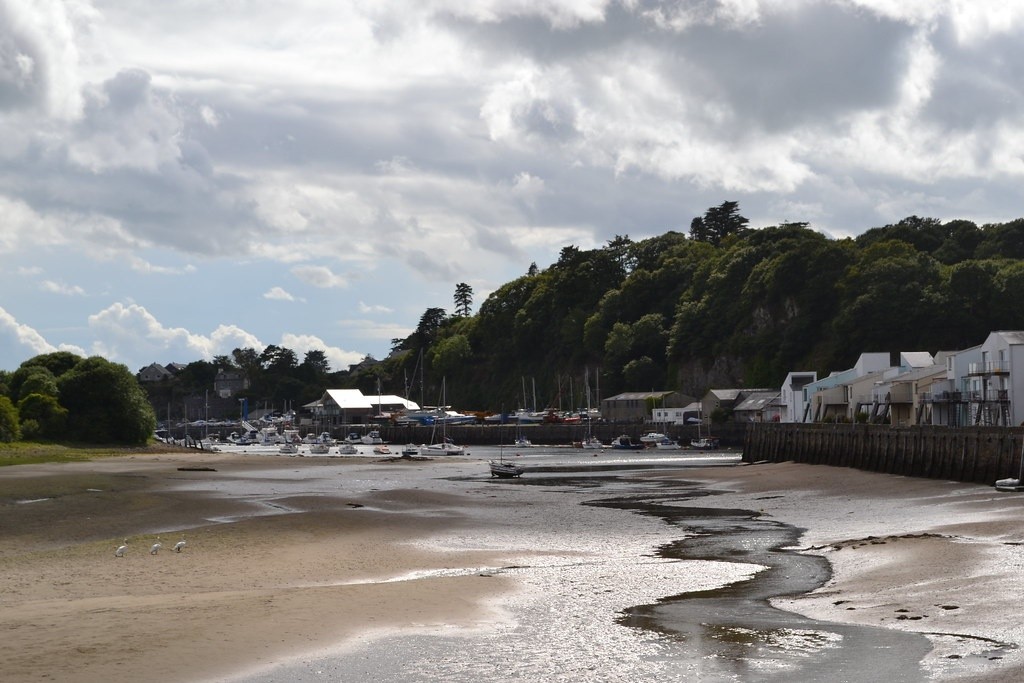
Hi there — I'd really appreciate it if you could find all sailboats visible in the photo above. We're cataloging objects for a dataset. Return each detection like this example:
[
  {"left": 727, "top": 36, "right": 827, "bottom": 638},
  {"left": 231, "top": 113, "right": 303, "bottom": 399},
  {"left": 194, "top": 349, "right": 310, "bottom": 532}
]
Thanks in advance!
[
  {"left": 487, "top": 402, "right": 526, "bottom": 478},
  {"left": 483, "top": 364, "right": 721, "bottom": 451},
  {"left": 162, "top": 346, "right": 479, "bottom": 455}
]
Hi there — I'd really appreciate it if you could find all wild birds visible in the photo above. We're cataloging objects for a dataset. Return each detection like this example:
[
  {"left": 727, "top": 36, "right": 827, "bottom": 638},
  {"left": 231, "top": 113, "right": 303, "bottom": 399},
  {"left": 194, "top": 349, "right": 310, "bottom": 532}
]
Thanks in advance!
[
  {"left": 170, "top": 534, "right": 187, "bottom": 553},
  {"left": 149, "top": 537, "right": 163, "bottom": 555},
  {"left": 113, "top": 539, "right": 129, "bottom": 558}
]
